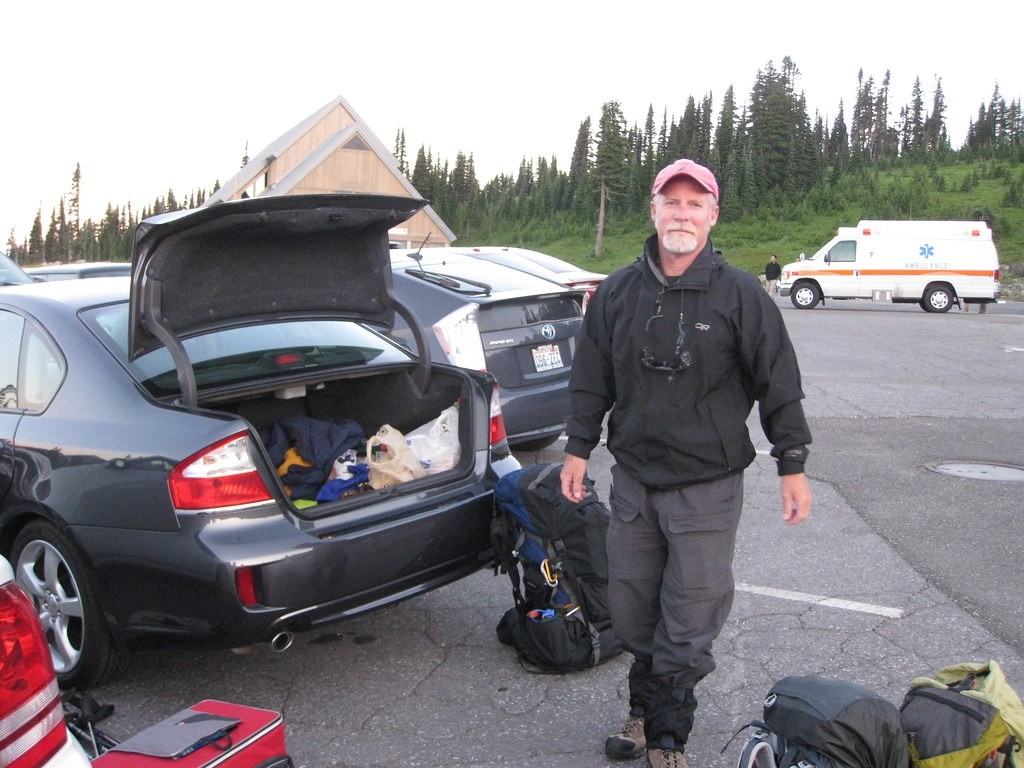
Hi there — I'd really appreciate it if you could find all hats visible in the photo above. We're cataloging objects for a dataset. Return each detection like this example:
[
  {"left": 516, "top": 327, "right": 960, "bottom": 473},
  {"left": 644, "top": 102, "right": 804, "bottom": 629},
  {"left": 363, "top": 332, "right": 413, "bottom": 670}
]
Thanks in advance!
[{"left": 650, "top": 158, "right": 718, "bottom": 203}]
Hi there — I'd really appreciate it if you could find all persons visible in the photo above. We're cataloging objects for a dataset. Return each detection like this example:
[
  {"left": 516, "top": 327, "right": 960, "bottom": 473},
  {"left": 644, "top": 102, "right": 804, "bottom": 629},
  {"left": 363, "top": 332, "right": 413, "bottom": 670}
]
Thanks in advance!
[
  {"left": 559, "top": 158, "right": 813, "bottom": 768},
  {"left": 765, "top": 255, "right": 781, "bottom": 300}
]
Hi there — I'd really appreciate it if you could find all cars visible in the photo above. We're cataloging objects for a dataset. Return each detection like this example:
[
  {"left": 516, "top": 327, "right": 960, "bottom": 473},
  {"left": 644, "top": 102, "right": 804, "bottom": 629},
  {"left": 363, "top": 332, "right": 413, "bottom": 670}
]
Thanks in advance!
[
  {"left": 364, "top": 233, "right": 612, "bottom": 458},
  {"left": 0, "top": 191, "right": 523, "bottom": 695}
]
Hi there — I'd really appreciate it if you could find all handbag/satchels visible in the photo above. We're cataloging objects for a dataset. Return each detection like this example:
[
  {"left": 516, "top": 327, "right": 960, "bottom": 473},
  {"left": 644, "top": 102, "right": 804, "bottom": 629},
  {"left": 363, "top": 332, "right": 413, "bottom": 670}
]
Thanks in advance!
[{"left": 366, "top": 405, "right": 460, "bottom": 491}]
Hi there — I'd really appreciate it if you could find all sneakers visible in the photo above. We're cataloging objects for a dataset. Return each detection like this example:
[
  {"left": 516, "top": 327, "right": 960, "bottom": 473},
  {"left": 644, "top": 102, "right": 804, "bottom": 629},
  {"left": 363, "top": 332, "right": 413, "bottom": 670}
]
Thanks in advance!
[
  {"left": 646, "top": 748, "right": 689, "bottom": 768},
  {"left": 604, "top": 714, "right": 647, "bottom": 757}
]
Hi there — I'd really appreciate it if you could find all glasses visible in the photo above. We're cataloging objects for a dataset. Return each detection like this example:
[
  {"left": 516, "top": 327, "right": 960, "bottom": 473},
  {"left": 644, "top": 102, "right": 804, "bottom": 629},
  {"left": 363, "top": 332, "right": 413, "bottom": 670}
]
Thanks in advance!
[{"left": 640, "top": 315, "right": 690, "bottom": 371}]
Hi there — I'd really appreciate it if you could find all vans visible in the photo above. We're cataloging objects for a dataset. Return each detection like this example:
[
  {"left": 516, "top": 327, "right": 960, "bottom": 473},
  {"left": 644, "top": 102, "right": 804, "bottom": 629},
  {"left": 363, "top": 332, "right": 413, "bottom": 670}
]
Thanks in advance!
[{"left": 775, "top": 220, "right": 1000, "bottom": 314}]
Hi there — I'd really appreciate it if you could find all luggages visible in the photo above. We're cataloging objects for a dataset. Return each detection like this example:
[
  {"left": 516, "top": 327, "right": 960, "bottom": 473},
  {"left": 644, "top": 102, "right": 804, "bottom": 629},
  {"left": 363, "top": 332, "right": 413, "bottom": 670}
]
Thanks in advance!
[{"left": 90, "top": 699, "right": 293, "bottom": 768}]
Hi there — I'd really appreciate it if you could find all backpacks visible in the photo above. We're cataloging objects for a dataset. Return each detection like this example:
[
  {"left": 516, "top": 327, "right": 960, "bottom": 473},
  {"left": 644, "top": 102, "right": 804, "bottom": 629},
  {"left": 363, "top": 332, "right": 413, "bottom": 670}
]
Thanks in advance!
[
  {"left": 491, "top": 461, "right": 626, "bottom": 674},
  {"left": 899, "top": 682, "right": 1021, "bottom": 768},
  {"left": 719, "top": 674, "right": 910, "bottom": 768}
]
